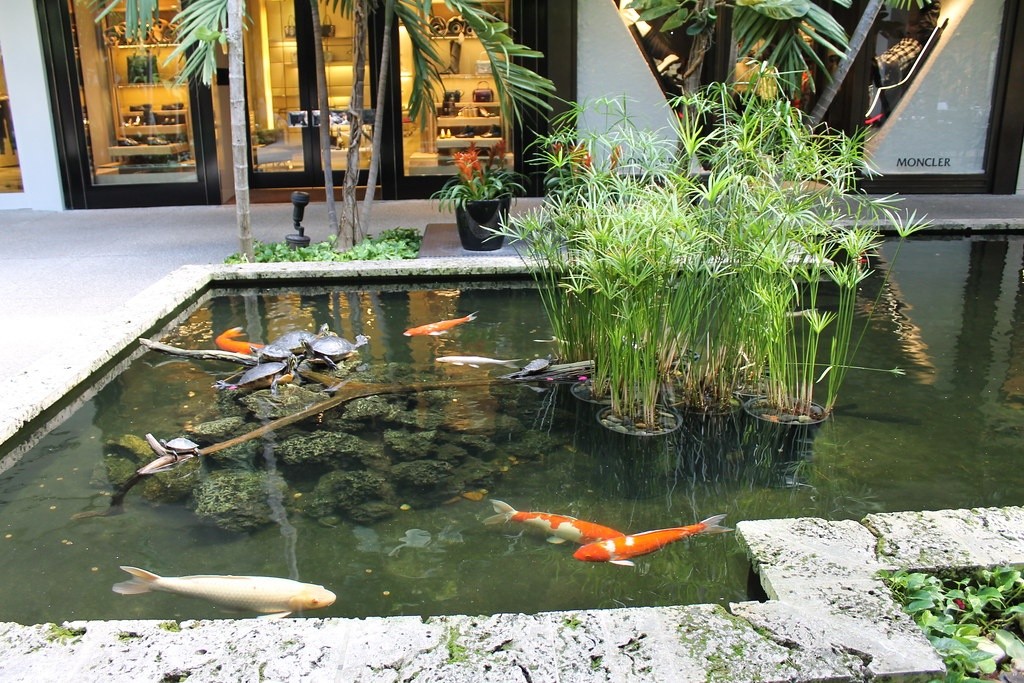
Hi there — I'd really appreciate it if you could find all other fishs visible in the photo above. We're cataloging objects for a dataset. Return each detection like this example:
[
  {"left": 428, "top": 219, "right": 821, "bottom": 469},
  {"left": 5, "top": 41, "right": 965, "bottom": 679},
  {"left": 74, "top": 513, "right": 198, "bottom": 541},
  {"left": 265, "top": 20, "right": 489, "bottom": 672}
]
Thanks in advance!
[
  {"left": 572, "top": 513, "right": 735, "bottom": 566},
  {"left": 404, "top": 311, "right": 479, "bottom": 337},
  {"left": 214, "top": 327, "right": 264, "bottom": 355},
  {"left": 436, "top": 356, "right": 522, "bottom": 369},
  {"left": 112, "top": 566, "right": 337, "bottom": 619},
  {"left": 482, "top": 499, "right": 625, "bottom": 545},
  {"left": 534, "top": 336, "right": 583, "bottom": 348}
]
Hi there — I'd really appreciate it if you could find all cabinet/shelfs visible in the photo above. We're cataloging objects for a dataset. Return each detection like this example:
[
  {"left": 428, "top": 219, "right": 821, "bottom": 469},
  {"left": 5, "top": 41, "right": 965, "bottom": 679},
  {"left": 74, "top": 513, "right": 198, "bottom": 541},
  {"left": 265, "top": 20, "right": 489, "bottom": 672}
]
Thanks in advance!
[
  {"left": 268, "top": 35, "right": 375, "bottom": 132},
  {"left": 424, "top": 0, "right": 513, "bottom": 168},
  {"left": 98, "top": 3, "right": 195, "bottom": 161}
]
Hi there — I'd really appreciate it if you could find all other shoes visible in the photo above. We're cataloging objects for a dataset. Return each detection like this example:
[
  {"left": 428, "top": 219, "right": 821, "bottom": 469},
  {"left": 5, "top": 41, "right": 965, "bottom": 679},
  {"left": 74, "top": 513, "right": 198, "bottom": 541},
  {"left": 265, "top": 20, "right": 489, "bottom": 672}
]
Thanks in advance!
[
  {"left": 146, "top": 136, "right": 170, "bottom": 145},
  {"left": 160, "top": 117, "right": 176, "bottom": 125},
  {"left": 440, "top": 129, "right": 452, "bottom": 138},
  {"left": 161, "top": 103, "right": 183, "bottom": 110},
  {"left": 455, "top": 125, "right": 474, "bottom": 138},
  {"left": 130, "top": 106, "right": 146, "bottom": 111},
  {"left": 481, "top": 125, "right": 502, "bottom": 138},
  {"left": 125, "top": 117, "right": 140, "bottom": 126},
  {"left": 117, "top": 139, "right": 138, "bottom": 145}
]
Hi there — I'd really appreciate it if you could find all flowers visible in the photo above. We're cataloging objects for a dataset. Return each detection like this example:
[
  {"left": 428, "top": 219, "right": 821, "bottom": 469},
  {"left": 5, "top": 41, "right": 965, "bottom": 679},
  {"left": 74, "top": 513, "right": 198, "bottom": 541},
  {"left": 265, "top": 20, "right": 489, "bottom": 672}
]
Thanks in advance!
[
  {"left": 544, "top": 139, "right": 628, "bottom": 206},
  {"left": 427, "top": 139, "right": 530, "bottom": 212}
]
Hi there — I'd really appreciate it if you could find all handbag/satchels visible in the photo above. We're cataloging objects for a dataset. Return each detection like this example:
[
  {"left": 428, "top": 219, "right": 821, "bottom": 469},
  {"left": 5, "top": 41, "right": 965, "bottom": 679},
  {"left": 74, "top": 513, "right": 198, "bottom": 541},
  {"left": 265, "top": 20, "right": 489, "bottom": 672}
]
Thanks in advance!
[
  {"left": 283, "top": 16, "right": 297, "bottom": 38},
  {"left": 443, "top": 89, "right": 464, "bottom": 103},
  {"left": 321, "top": 15, "right": 336, "bottom": 38},
  {"left": 126, "top": 49, "right": 161, "bottom": 83},
  {"left": 440, "top": 103, "right": 458, "bottom": 118},
  {"left": 330, "top": 129, "right": 345, "bottom": 150},
  {"left": 472, "top": 81, "right": 494, "bottom": 102}
]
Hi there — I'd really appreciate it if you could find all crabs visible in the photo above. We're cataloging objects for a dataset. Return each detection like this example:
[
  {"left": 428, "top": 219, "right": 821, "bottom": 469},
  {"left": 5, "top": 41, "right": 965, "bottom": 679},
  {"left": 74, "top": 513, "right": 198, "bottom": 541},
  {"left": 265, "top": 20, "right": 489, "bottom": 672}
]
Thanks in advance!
[{"left": 160, "top": 437, "right": 201, "bottom": 461}]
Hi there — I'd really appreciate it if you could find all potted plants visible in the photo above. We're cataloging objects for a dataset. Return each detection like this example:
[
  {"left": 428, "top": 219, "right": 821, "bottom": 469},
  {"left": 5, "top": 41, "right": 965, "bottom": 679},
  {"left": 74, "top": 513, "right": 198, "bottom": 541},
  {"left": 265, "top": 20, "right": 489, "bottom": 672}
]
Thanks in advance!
[{"left": 478, "top": 55, "right": 933, "bottom": 503}]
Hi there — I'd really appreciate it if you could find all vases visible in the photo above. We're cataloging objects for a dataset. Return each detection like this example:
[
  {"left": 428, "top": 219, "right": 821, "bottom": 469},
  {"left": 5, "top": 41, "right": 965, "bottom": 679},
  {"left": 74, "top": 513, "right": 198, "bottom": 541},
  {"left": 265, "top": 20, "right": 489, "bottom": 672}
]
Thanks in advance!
[{"left": 453, "top": 195, "right": 511, "bottom": 250}]
